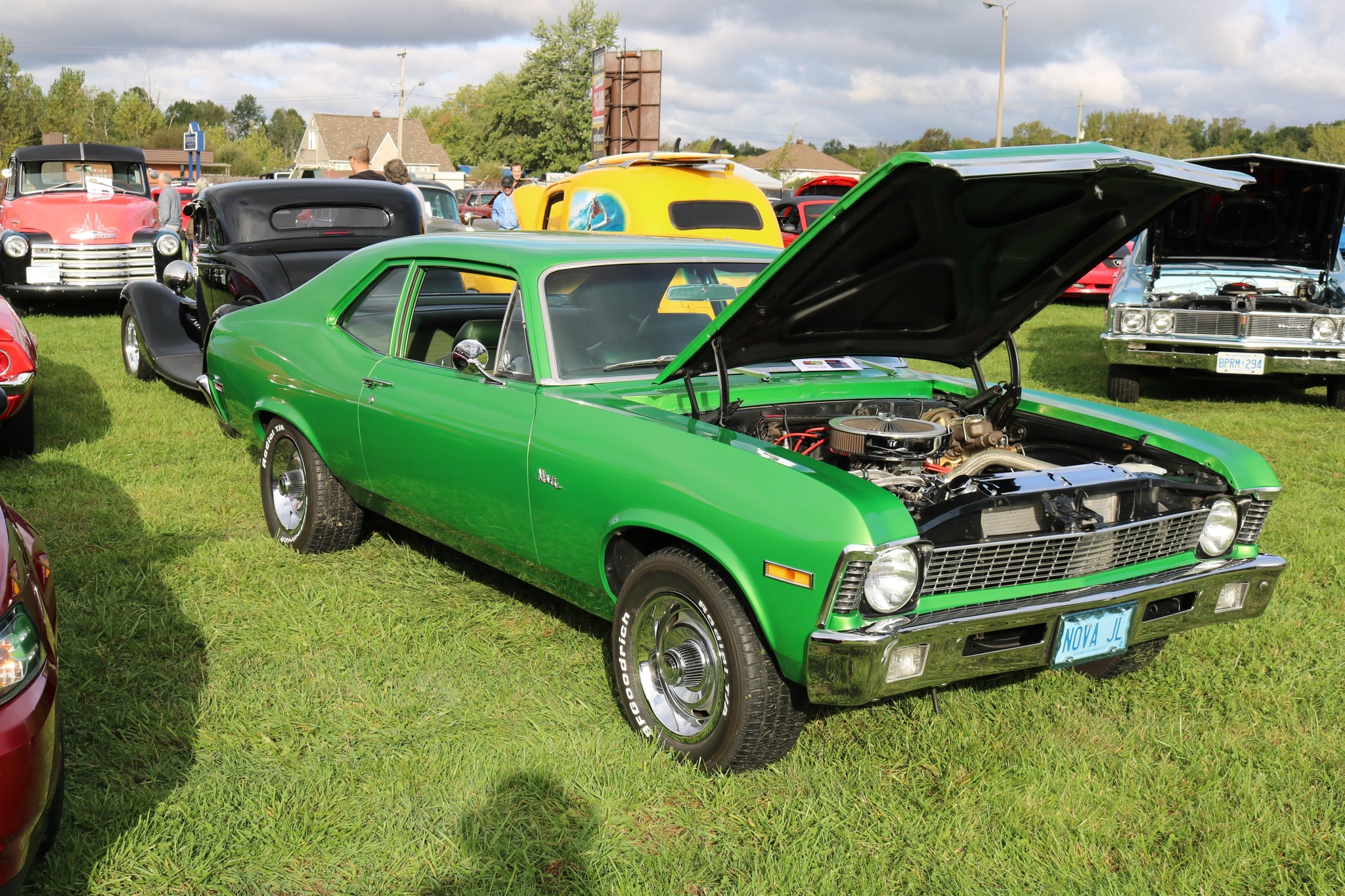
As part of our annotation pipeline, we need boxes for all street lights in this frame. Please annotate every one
[398,82,427,160]
[981,1,1007,149]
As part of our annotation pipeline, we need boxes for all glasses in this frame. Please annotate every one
[349,156,356,160]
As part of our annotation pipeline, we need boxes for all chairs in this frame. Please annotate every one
[595,312,713,362]
[456,319,574,374]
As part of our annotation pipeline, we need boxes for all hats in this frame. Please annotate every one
[501,175,513,187]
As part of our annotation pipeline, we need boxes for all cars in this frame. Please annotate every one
[1058,155,1345,409]
[195,145,1286,777]
[0,494,62,892]
[2,141,183,308]
[1,294,36,461]
[119,152,860,394]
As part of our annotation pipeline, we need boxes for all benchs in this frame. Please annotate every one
[349,306,591,367]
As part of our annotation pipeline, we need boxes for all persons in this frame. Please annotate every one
[510,162,531,190]
[158,172,182,235]
[193,178,209,193]
[491,176,521,231]
[330,145,388,226]
[585,196,610,230]
[384,158,427,232]
